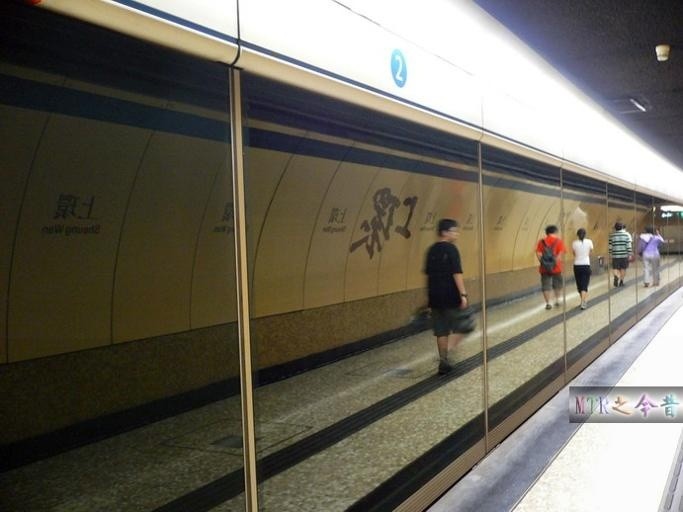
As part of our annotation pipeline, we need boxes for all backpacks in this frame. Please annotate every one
[540,238,559,273]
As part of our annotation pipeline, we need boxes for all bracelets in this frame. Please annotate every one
[460,293,468,297]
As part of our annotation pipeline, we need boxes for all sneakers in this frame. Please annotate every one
[614,276,624,287]
[438,353,457,373]
[546,300,587,310]
[644,282,660,287]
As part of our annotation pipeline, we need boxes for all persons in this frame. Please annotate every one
[572,228,595,310]
[609,222,633,288]
[638,224,665,287]
[535,224,567,310]
[420,218,478,374]
